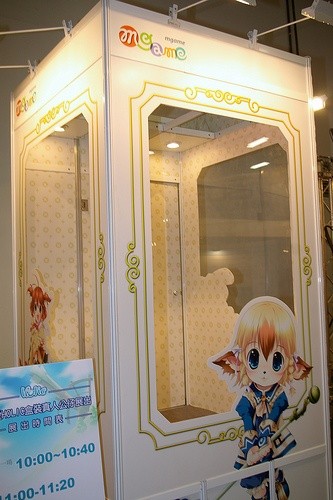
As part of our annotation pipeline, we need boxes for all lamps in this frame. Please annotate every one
[168,0,258,26]
[245,1,333,49]
